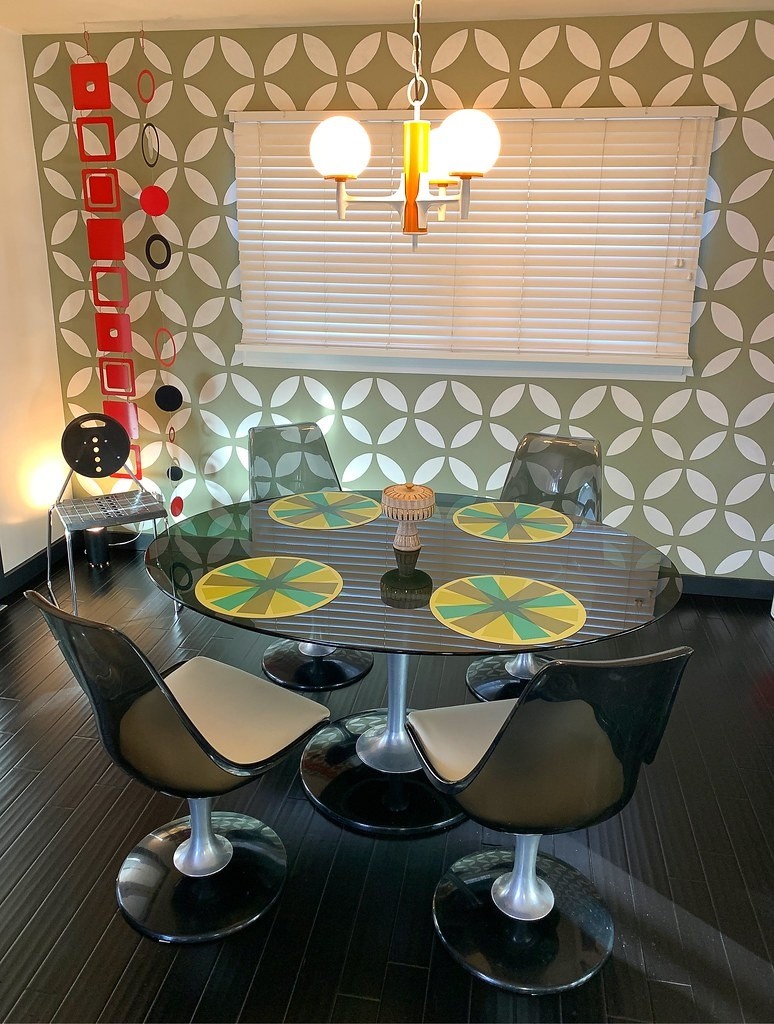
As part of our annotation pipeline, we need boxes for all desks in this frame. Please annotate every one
[144,491,682,839]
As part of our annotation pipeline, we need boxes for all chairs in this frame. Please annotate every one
[24,590,331,945]
[405,646,695,996]
[47,414,184,616]
[466,432,602,702]
[248,423,374,691]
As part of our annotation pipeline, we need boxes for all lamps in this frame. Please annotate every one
[309,1,500,248]
[83,527,110,568]
